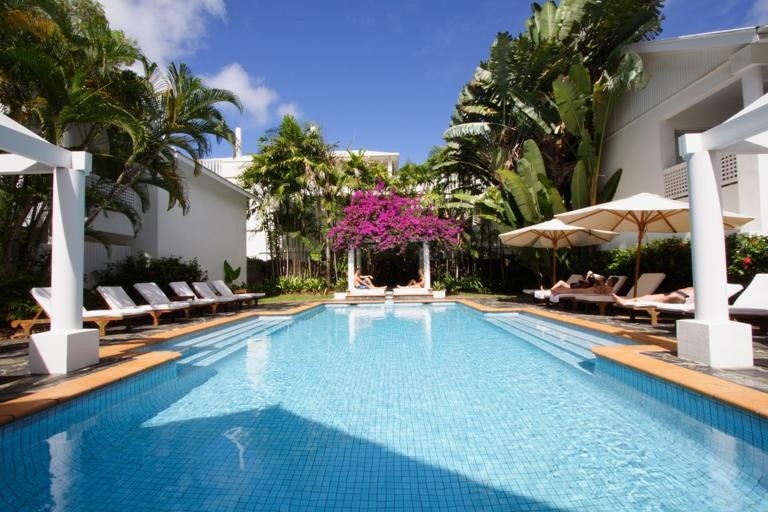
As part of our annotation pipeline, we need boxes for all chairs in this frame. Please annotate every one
[8,276,267,338]
[392,275,426,294]
[518,268,768,345]
[344,273,386,295]
[347,308,386,343]
[394,308,432,345]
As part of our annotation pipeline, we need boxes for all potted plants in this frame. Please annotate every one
[333,285,347,300]
[430,280,446,299]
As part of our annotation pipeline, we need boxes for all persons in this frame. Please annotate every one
[354,268,375,288]
[551,273,619,295]
[610,286,694,306]
[397,268,425,288]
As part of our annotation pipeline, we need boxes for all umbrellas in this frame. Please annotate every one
[554,191,756,301]
[498,218,620,288]
